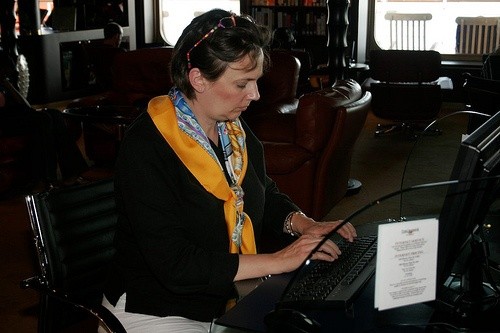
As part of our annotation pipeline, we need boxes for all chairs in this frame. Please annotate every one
[456,17,500,54]
[463,72,500,135]
[385,13,432,51]
[14,177,127,333]
[274,49,323,98]
[364,50,443,144]
[69,45,114,96]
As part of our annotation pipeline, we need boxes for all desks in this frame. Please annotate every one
[63,105,145,180]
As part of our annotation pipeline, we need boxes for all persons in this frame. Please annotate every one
[92,23,127,90]
[0,73,113,184]
[98,9,358,333]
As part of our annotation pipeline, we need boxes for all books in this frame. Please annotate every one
[251,0,327,36]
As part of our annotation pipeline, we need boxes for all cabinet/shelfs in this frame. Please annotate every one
[240,0,358,64]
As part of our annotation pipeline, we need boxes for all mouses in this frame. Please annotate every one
[264,310,319,333]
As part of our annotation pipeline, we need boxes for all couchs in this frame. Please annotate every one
[0,47,373,221]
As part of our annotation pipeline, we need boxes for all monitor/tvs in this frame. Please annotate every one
[423,113,500,327]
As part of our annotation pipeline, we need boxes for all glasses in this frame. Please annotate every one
[185,14,256,72]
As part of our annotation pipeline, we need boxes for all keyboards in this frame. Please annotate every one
[281,236,380,306]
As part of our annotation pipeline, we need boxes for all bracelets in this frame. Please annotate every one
[284,211,306,237]
